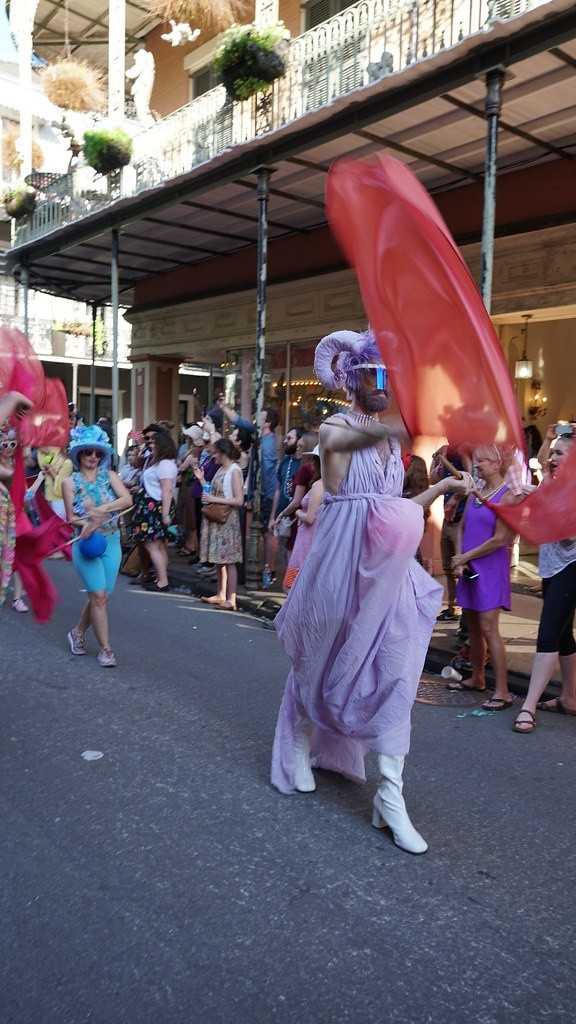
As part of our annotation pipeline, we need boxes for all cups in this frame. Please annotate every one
[441,666,462,682]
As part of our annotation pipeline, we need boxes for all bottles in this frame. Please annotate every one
[262,563,271,590]
[202,481,212,504]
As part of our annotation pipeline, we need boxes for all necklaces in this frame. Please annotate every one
[476,482,506,504]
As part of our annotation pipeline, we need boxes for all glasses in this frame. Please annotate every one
[143,434,157,440]
[79,449,104,459]
[283,435,297,441]
[1,440,17,450]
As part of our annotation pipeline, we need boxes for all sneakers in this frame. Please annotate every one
[68,629,86,654]
[97,649,118,667]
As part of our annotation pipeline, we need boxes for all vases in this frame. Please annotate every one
[221,50,284,101]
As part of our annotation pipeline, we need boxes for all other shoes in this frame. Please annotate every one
[529,585,543,592]
[49,550,66,559]
[128,543,291,630]
[12,598,29,612]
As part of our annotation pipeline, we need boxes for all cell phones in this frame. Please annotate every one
[555,424,573,435]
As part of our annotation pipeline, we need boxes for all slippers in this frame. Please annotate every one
[436,607,576,733]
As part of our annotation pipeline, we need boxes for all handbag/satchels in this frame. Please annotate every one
[276,516,292,537]
[202,466,244,525]
[119,543,143,578]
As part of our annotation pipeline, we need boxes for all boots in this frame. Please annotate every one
[291,713,316,793]
[372,753,429,855]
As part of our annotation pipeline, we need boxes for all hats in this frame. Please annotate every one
[301,442,320,457]
[68,426,113,467]
[181,425,205,446]
[141,419,175,434]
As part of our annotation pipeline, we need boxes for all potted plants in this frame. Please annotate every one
[0,185,36,218]
[82,129,132,177]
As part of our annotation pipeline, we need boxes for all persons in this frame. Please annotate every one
[0,391,113,612]
[61,425,133,666]
[270,330,478,852]
[402,406,576,733]
[120,397,324,630]
[125,49,154,128]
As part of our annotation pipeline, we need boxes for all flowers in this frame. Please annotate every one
[209,20,289,102]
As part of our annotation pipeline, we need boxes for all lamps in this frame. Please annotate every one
[515,313,534,380]
[527,377,547,421]
